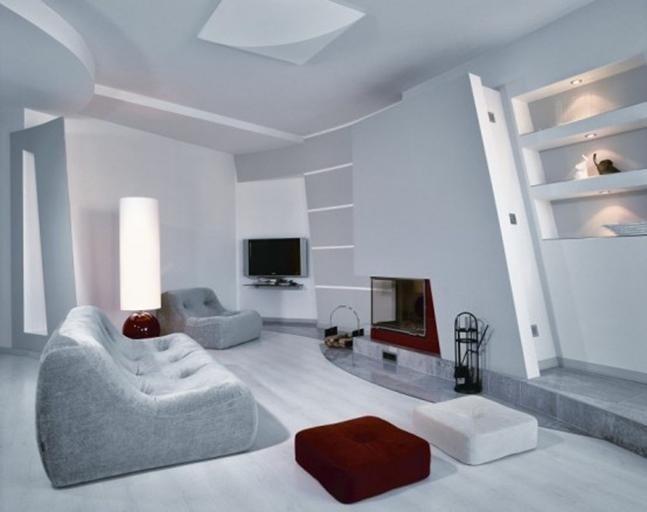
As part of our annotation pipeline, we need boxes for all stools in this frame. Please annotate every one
[295,416,430,504]
[413,395,538,466]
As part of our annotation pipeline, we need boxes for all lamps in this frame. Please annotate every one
[118,195,162,339]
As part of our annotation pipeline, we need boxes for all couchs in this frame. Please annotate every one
[156,288,262,350]
[34,305,257,489]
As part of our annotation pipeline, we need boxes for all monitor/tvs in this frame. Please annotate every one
[372,276,426,337]
[243,238,307,290]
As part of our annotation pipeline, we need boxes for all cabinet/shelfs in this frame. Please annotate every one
[504,31,647,241]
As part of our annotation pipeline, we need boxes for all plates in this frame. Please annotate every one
[602,222,647,237]
